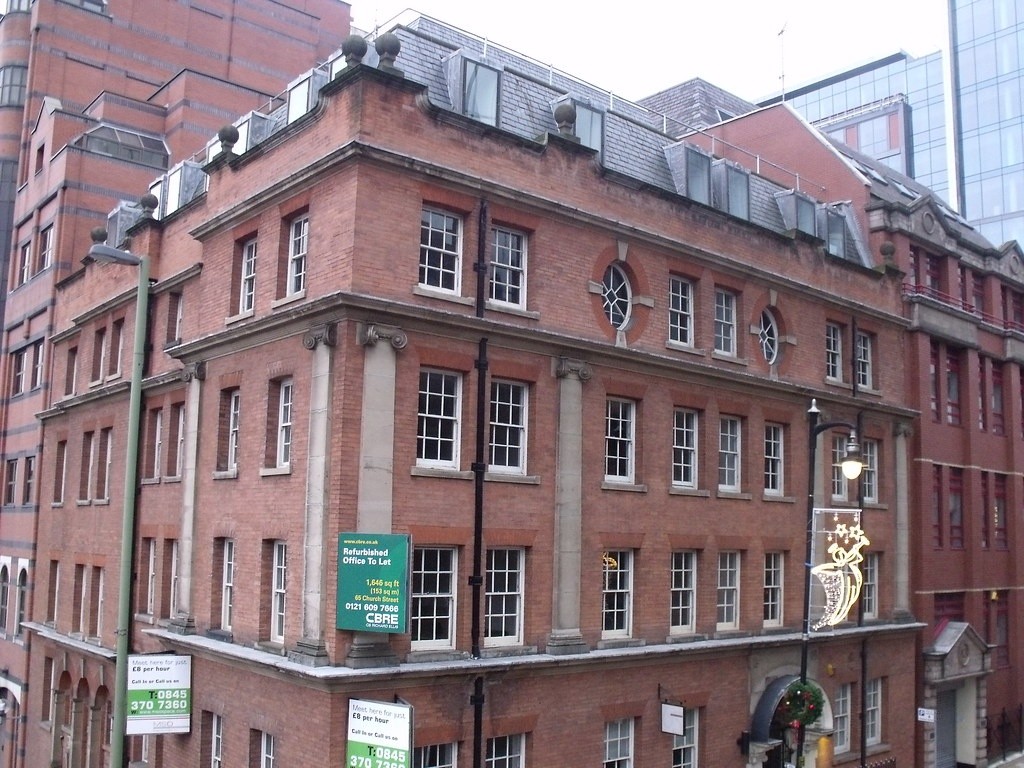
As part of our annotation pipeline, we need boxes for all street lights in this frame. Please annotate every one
[796,399,863,768]
[88,244,151,768]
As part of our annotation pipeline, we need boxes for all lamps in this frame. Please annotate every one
[810,417,861,481]
